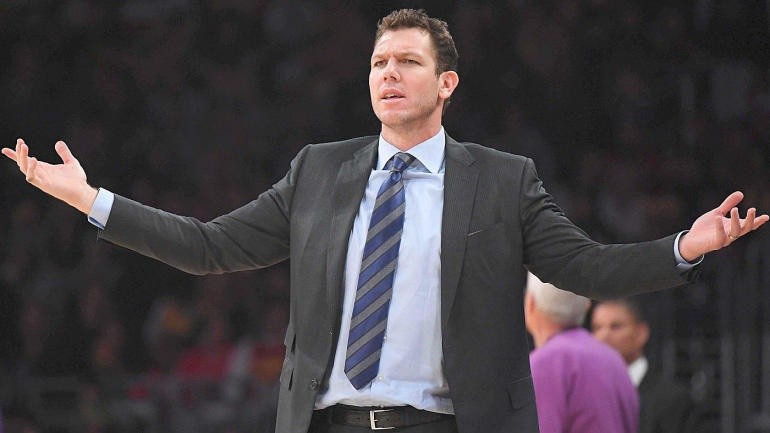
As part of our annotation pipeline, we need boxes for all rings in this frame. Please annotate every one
[727,232,738,241]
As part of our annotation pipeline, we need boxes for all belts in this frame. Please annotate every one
[313,406,455,429]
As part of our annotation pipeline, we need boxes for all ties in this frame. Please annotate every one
[344,153,416,390]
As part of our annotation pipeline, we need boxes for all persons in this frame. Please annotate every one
[525,271,642,433]
[2,9,770,433]
[591,298,685,433]
